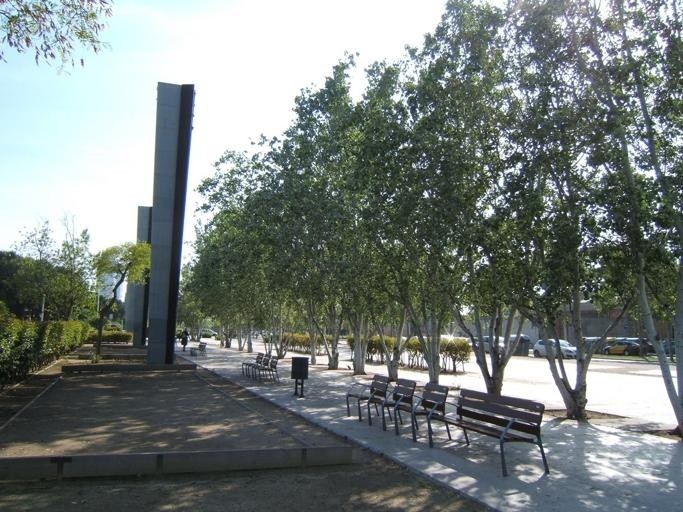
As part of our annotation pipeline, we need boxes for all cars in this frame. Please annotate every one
[534,339,578,358]
[605,340,640,355]
[195,329,218,336]
[467,337,478,349]
[483,336,505,353]
[509,334,533,349]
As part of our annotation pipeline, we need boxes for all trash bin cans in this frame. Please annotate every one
[292,357,309,398]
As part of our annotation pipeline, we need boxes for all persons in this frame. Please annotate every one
[178,327,191,352]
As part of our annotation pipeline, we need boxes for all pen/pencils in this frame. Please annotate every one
[504,334,530,356]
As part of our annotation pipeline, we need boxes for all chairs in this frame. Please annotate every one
[190,341,207,356]
[242,353,280,382]
[346,375,549,476]
